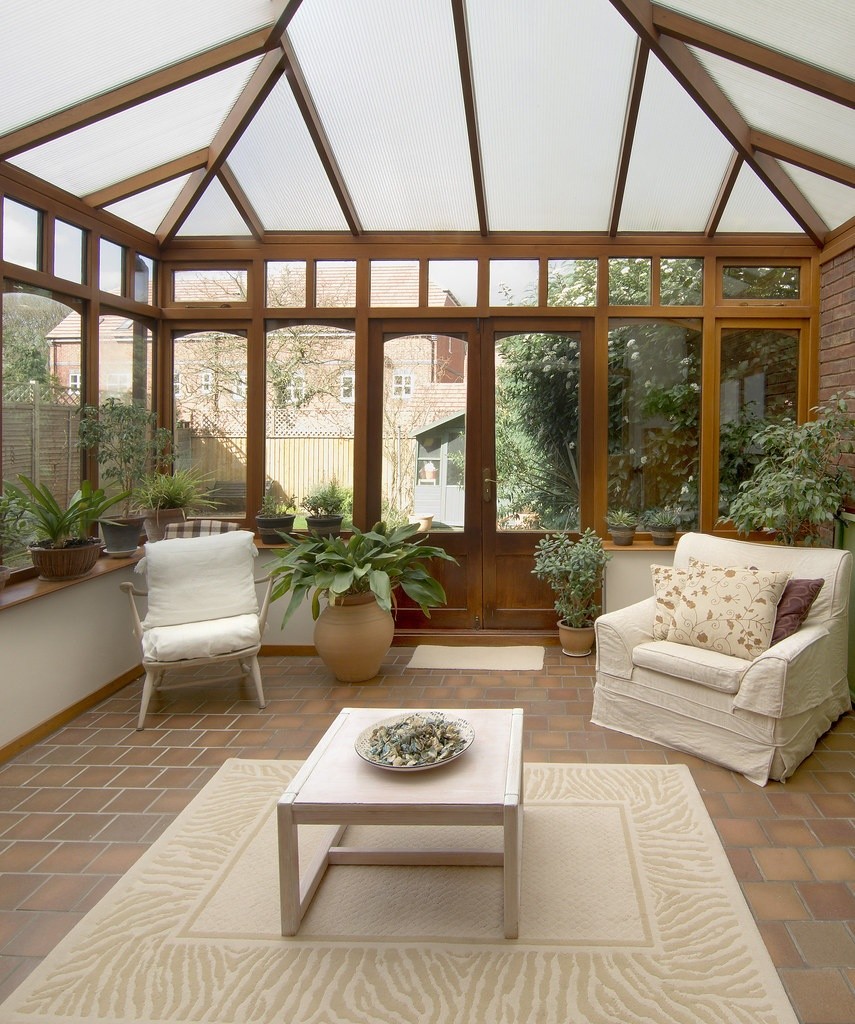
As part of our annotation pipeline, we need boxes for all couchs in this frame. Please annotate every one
[590,533,852,787]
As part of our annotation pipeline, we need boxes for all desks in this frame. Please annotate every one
[418,479,436,485]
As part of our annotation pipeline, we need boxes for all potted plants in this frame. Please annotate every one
[255,493,298,544]
[130,462,226,543]
[602,508,639,546]
[420,462,437,479]
[260,517,460,682]
[639,506,684,546]
[299,482,352,541]
[1,473,131,581]
[0,491,32,592]
[530,527,614,656]
[70,397,184,559]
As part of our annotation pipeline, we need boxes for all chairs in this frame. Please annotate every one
[119,520,275,731]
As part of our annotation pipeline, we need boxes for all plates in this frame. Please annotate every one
[355,711,474,772]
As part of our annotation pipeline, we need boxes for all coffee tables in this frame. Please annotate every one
[277,708,524,939]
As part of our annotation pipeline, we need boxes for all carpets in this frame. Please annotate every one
[0,757,800,1024]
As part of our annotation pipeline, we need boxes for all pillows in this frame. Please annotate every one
[134,531,259,630]
[770,578,825,648]
[651,563,758,641]
[667,556,793,662]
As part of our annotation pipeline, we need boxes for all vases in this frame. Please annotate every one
[409,513,434,531]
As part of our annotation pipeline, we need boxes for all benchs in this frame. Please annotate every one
[205,481,275,510]
